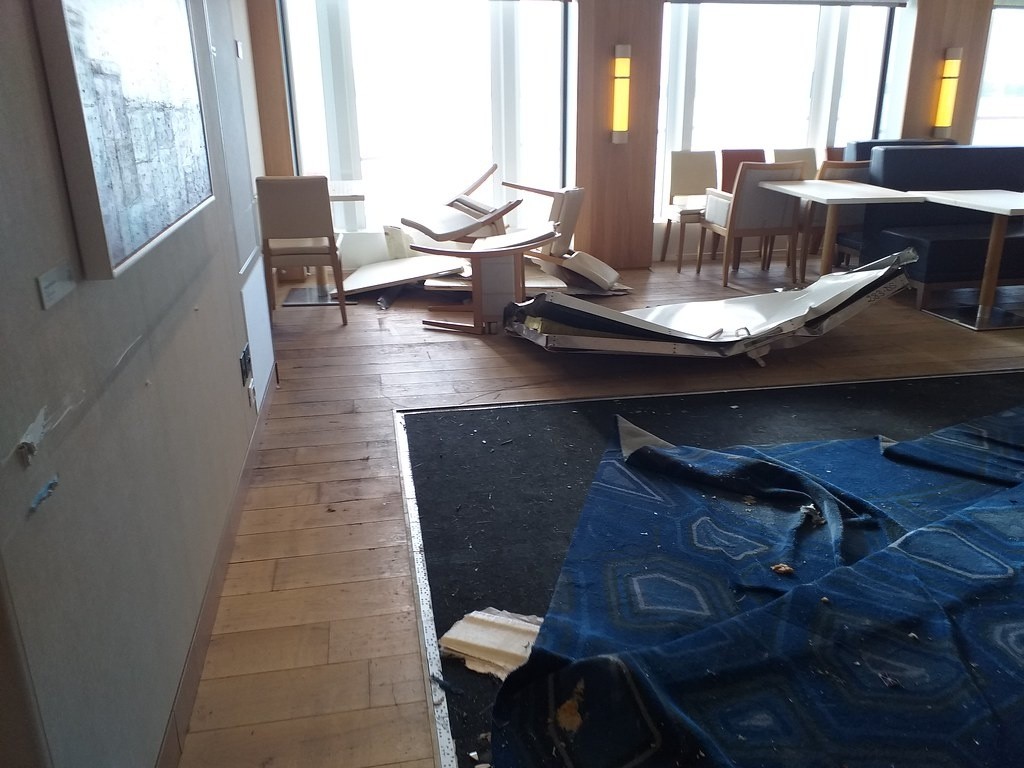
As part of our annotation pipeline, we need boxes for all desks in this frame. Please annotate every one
[910,186,1024,334]
[759,178,922,289]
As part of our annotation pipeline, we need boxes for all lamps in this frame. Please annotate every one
[608,39,632,147]
[934,43,965,138]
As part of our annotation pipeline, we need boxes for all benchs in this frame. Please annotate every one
[840,140,1024,313]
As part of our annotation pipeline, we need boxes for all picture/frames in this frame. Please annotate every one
[35,0,220,289]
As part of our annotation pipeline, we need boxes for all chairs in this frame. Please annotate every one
[657,149,738,274]
[825,146,843,161]
[251,158,624,338]
[720,146,775,274]
[695,158,808,288]
[771,145,818,263]
[798,158,872,278]
